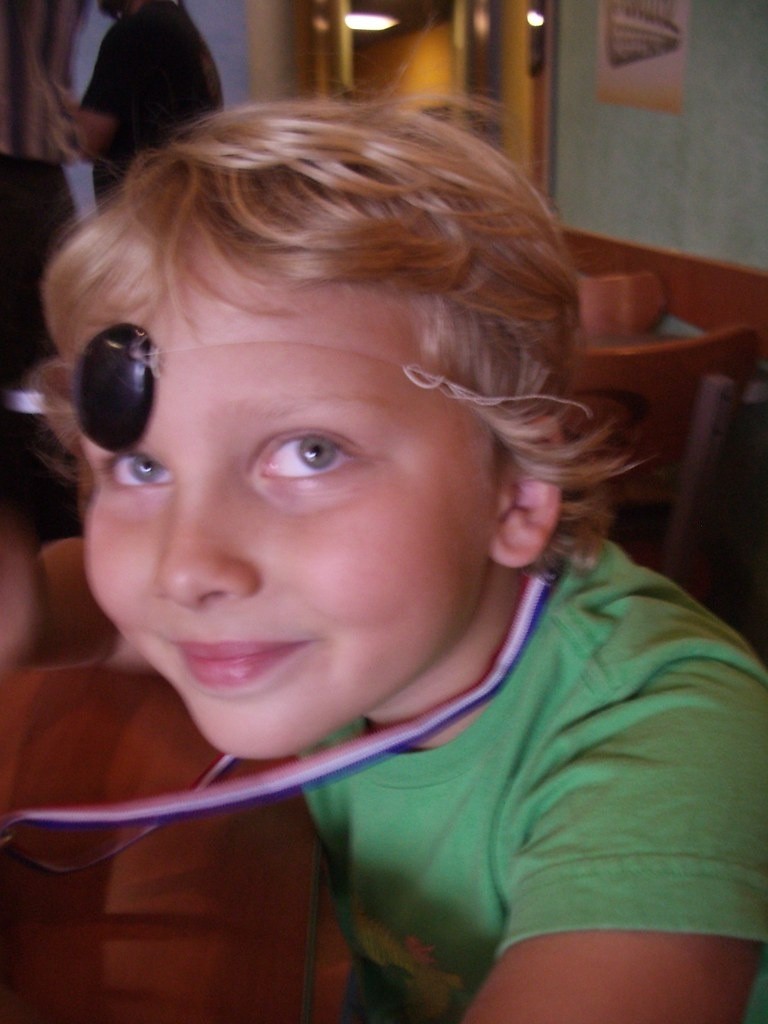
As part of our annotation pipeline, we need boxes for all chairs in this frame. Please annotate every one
[568,324,761,602]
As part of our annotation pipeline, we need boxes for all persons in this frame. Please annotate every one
[0,101,768,1024]
[0,0,222,542]
